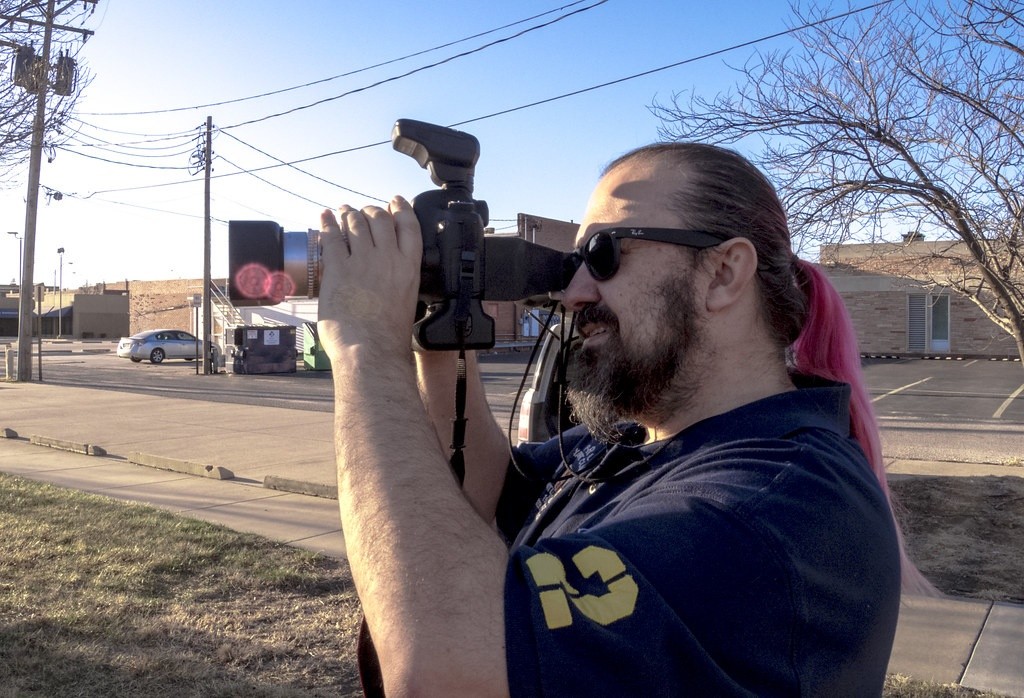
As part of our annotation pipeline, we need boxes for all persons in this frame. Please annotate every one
[317,141,901,698]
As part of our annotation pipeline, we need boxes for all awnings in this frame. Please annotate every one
[32,305,54,317]
[0,309,19,318]
[46,306,73,318]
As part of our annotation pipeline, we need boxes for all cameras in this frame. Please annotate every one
[228,117,578,353]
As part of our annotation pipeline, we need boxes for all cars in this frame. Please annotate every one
[116,327,221,364]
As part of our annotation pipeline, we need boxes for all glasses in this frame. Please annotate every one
[570,228,770,282]
